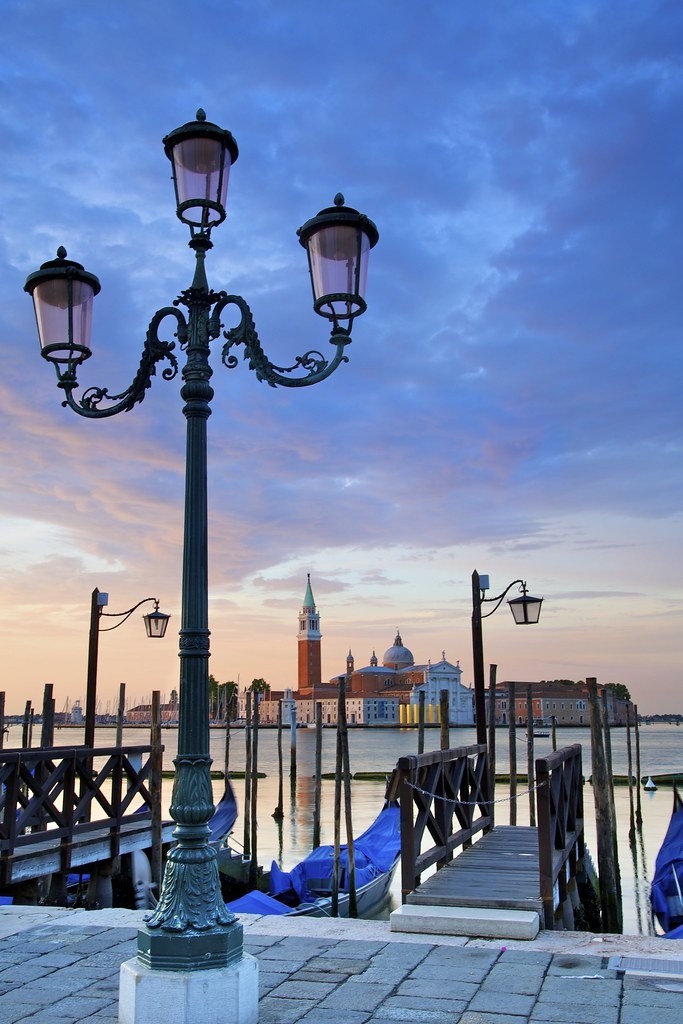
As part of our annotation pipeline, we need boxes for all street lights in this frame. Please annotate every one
[471,569,539,808]
[23,101,382,972]
[83,586,171,792]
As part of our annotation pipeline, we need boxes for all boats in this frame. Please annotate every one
[525,731,550,737]
[643,776,658,791]
